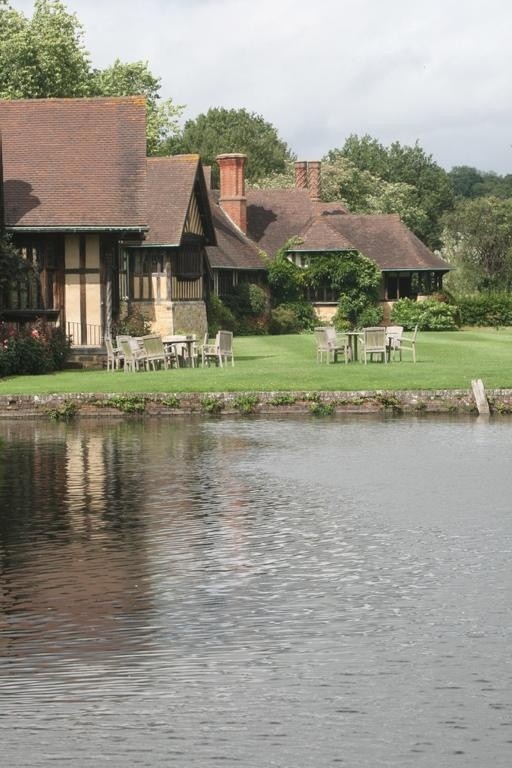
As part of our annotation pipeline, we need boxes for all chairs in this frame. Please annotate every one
[314,323,419,365]
[104,330,234,371]
